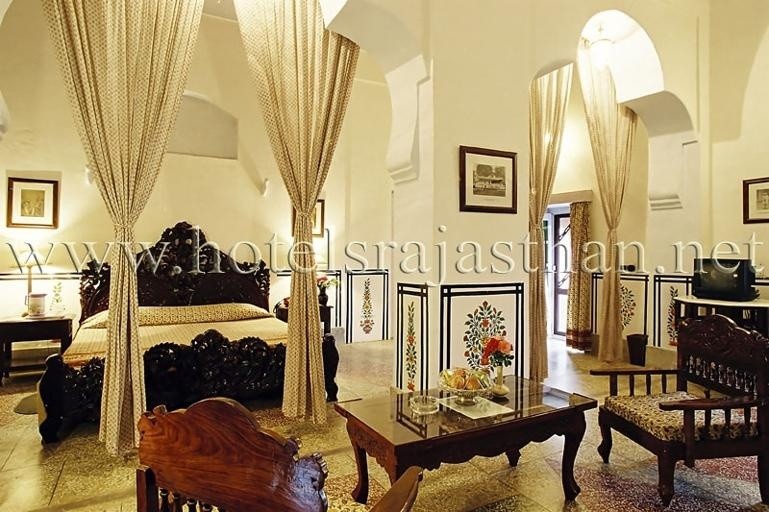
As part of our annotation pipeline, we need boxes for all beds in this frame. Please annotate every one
[35,222,340,448]
[334,374,597,504]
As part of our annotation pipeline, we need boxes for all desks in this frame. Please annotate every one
[670,295,769,339]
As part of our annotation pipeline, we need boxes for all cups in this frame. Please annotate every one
[30,298,42,315]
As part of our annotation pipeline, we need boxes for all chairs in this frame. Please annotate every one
[590,314,769,506]
[136,396,423,512]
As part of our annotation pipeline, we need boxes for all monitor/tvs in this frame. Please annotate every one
[692,259,760,302]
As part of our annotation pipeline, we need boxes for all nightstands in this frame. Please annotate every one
[276,297,333,339]
[1,317,72,389]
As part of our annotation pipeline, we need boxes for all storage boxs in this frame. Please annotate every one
[11,339,62,360]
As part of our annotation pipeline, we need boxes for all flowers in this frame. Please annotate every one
[316,274,338,289]
[481,334,514,367]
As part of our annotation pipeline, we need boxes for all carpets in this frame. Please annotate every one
[336,382,362,402]
[14,393,39,414]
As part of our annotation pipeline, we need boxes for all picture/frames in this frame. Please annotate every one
[7,177,58,228]
[292,199,323,236]
[458,146,516,214]
[743,177,769,224]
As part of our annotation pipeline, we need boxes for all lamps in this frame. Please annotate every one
[582,25,612,56]
[8,251,53,317]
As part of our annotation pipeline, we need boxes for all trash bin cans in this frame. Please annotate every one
[627,334,649,367]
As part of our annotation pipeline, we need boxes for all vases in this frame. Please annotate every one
[318,288,330,304]
[491,365,510,396]
